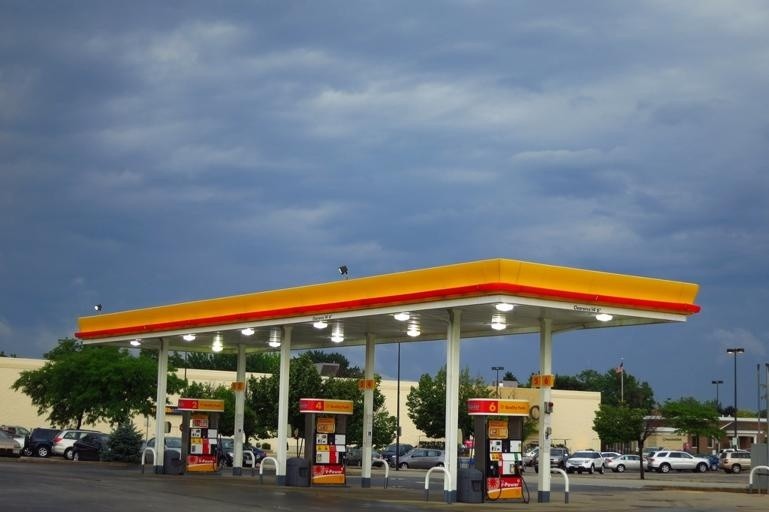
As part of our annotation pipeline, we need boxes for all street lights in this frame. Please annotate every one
[726,347,743,447]
[711,380,724,401]
[491,366,504,398]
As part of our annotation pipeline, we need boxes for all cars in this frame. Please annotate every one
[346,446,382,467]
[218,437,254,468]
[146,434,181,464]
[0,430,21,457]
[243,444,265,463]
[72,433,111,460]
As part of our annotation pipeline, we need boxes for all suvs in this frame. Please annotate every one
[392,447,444,470]
[521,446,753,476]
[0,425,32,456]
[52,428,103,460]
[30,427,61,458]
[381,443,413,463]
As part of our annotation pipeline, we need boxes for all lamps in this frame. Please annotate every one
[339,266,349,280]
[94,304,102,315]
